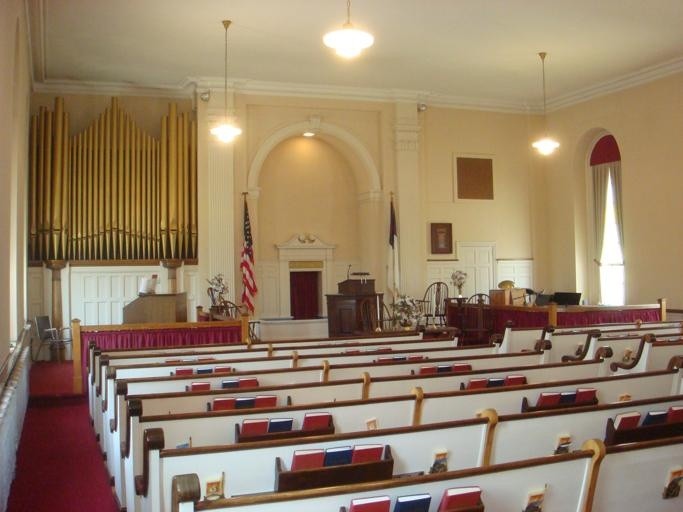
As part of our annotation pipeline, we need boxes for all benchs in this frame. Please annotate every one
[85,320,683,512]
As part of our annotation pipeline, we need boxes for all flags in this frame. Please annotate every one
[387,198,402,298]
[240,195,259,317]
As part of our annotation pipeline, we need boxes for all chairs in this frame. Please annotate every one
[362,297,394,334]
[218,301,260,344]
[33,315,72,366]
[422,281,449,327]
[460,294,494,347]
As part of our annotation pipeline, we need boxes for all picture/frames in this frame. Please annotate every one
[452,153,497,205]
[426,218,458,261]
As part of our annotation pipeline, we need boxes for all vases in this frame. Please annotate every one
[458,285,462,298]
[213,291,221,307]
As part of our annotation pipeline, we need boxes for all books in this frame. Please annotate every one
[439,485,482,512]
[324,445,352,468]
[352,444,384,463]
[290,448,326,470]
[612,409,642,431]
[665,405,683,423]
[213,394,277,411]
[377,355,417,362]
[175,366,234,376]
[378,347,392,351]
[347,494,391,512]
[419,363,472,374]
[467,374,525,389]
[165,356,216,363]
[191,378,257,391]
[241,412,330,436]
[393,492,433,512]
[534,387,597,407]
[346,349,360,353]
[639,410,670,427]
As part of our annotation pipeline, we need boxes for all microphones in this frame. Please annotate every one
[347,264,352,279]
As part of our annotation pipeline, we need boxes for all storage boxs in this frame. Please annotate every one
[489,287,526,305]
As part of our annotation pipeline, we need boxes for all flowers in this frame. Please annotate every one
[391,293,421,321]
[205,272,228,295]
[451,270,467,287]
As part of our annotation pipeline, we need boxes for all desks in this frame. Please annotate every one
[444,297,468,327]
[209,306,231,322]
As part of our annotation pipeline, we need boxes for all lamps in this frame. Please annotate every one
[532,53,561,156]
[209,21,242,145]
[321,0,376,62]
